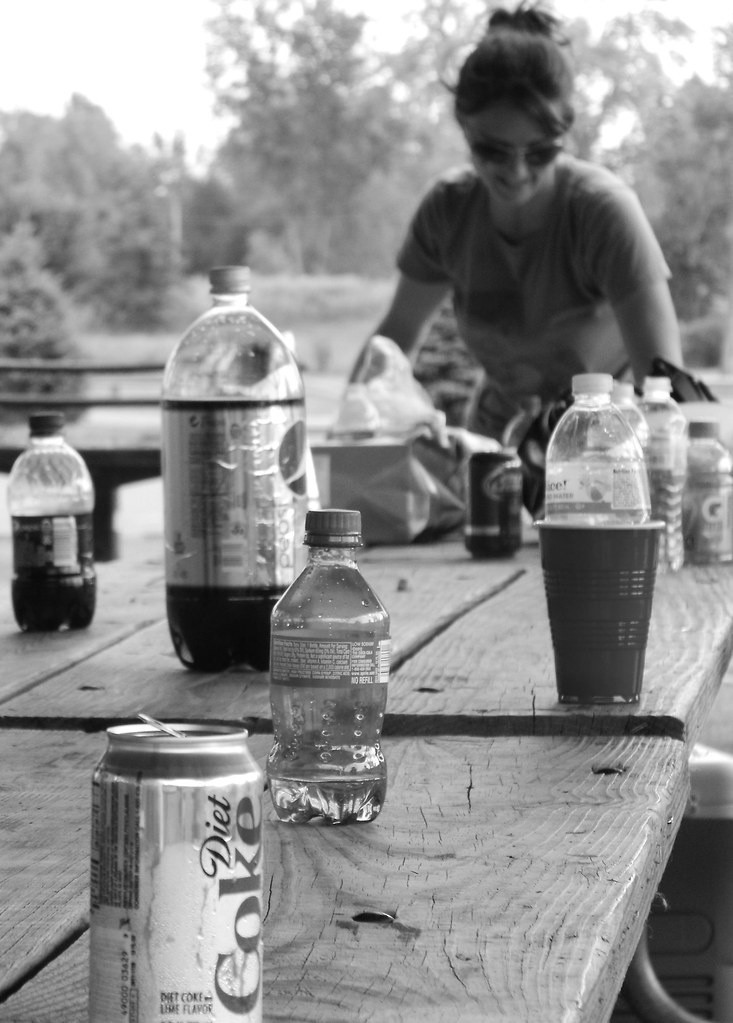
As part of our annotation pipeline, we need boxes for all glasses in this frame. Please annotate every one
[466,140,563,167]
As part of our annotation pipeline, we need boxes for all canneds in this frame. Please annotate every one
[463,448,525,556]
[90,712,266,1023]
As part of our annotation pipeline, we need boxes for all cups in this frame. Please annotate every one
[537,520,665,703]
[310,442,434,544]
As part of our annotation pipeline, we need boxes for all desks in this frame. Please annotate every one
[0,526,732,1023]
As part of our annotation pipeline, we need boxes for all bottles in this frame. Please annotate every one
[268,509,389,825]
[638,375,685,573]
[7,411,97,630]
[683,422,733,564]
[163,266,306,670]
[546,373,653,523]
[502,396,541,457]
[610,380,649,465]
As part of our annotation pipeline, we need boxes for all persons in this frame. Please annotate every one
[350,0,682,444]
